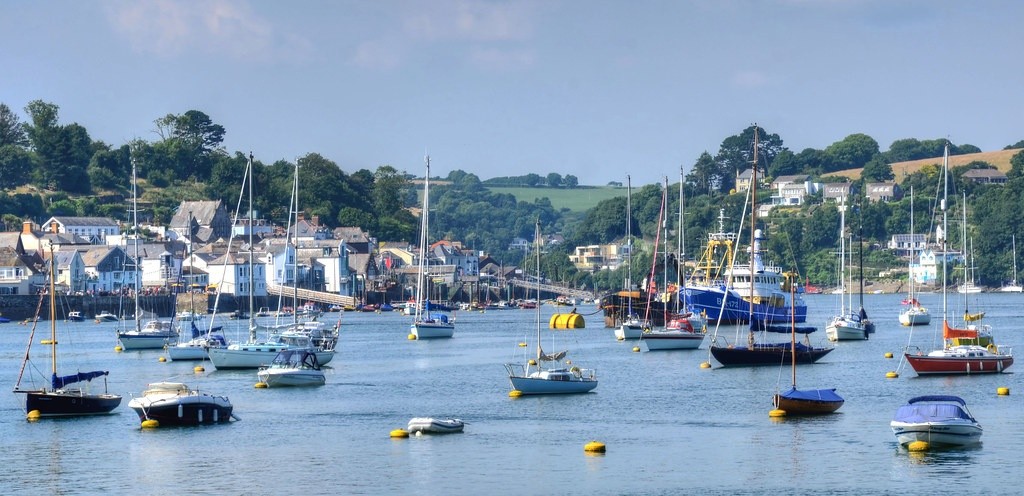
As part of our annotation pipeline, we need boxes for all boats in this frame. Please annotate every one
[0,315,13,324]
[890,394,984,450]
[256,346,328,389]
[66,311,85,323]
[24,315,43,323]
[407,415,464,434]
[175,310,205,322]
[126,380,234,429]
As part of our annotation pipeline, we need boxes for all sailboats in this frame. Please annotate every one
[904,136,1014,378]
[11,238,124,419]
[164,210,231,363]
[406,155,457,340]
[853,226,878,334]
[93,266,118,322]
[501,215,600,395]
[597,162,808,351]
[263,158,341,350]
[955,236,989,295]
[113,157,182,351]
[824,187,868,343]
[201,150,335,371]
[999,235,1024,293]
[896,184,933,328]
[706,120,836,366]
[948,189,994,348]
[770,268,846,415]
[228,233,593,320]
